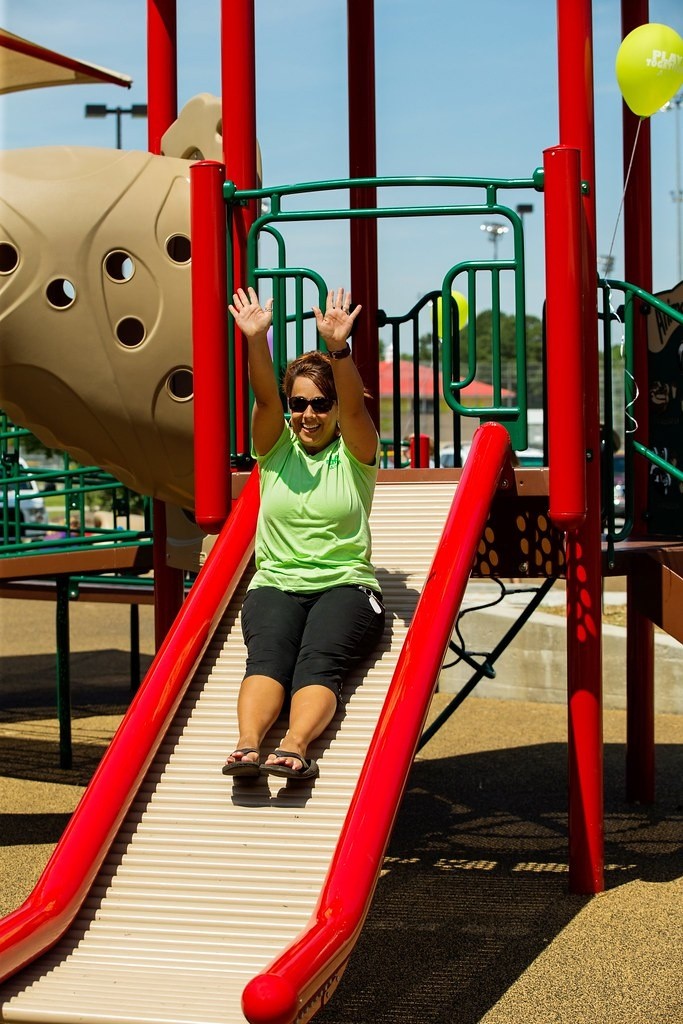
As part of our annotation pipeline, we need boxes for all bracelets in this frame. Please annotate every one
[327,342,351,360]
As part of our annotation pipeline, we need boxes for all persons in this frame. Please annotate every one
[27,512,101,542]
[220,285,386,781]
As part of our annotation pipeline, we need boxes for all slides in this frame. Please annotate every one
[0,414,514,1024]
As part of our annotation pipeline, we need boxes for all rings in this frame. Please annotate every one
[343,308,349,312]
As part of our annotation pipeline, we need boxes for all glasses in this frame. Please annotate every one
[288,396,335,413]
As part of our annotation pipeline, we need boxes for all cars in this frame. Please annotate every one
[0,456,48,538]
[437,442,544,466]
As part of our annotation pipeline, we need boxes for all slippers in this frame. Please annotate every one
[259,750,319,779]
[222,748,262,776]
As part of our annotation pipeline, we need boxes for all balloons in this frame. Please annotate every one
[614,23,683,116]
[431,291,468,337]
